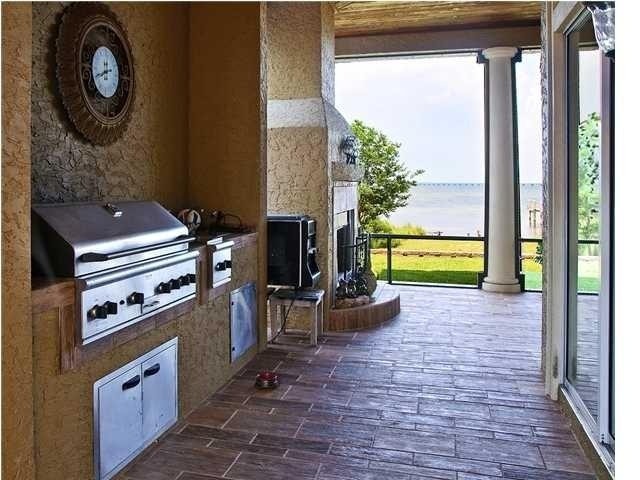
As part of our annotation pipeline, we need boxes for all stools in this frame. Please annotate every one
[269,288,326,353]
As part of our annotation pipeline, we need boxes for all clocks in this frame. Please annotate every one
[50,2,136,148]
